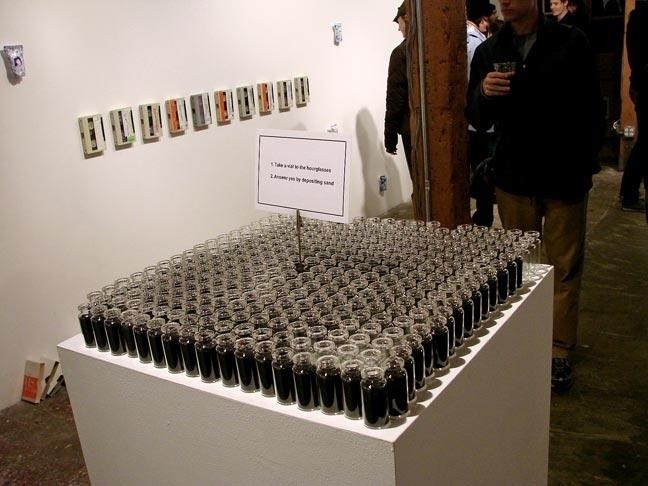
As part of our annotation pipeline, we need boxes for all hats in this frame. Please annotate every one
[393,2,406,24]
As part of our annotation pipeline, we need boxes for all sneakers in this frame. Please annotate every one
[551,349,573,392]
[622,198,647,213]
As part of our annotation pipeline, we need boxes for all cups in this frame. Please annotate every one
[495,61,515,81]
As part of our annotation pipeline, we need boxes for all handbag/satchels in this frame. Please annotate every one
[472,157,495,199]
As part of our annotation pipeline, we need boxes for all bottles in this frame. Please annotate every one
[77,214,541,429]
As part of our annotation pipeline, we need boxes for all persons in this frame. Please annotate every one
[466,0,577,228]
[385,0,411,179]
[466,1,603,394]
[619,0,648,212]
